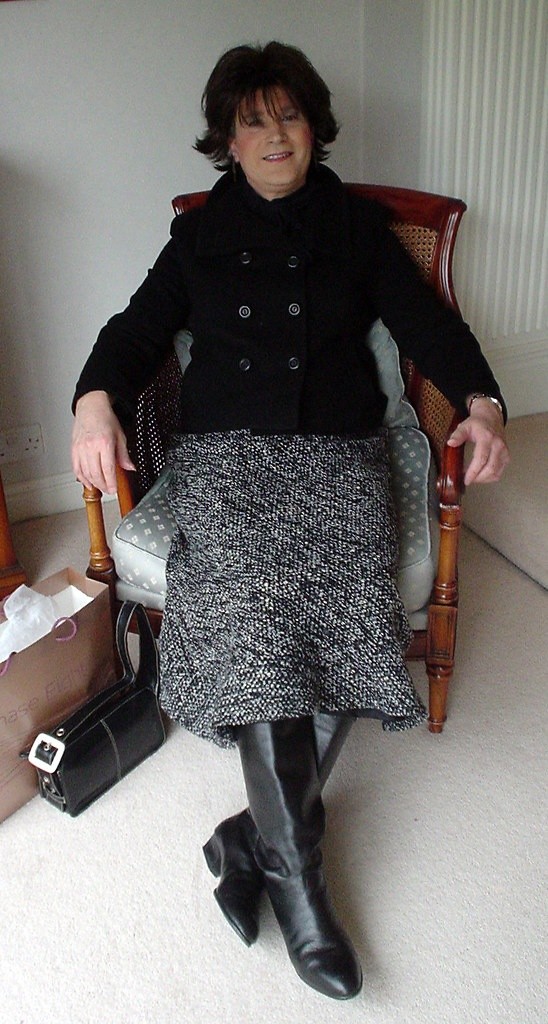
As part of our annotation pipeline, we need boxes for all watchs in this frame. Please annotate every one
[467,394,502,418]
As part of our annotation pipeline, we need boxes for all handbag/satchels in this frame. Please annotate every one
[0,567,118,824]
[20,599,166,818]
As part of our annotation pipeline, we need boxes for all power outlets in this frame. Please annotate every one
[0,423,43,466]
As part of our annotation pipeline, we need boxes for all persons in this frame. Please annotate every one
[71,42,510,1001]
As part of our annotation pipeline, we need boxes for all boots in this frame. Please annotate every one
[202,711,356,947]
[235,715,363,1000]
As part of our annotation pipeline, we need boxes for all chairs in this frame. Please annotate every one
[80,181,472,733]
